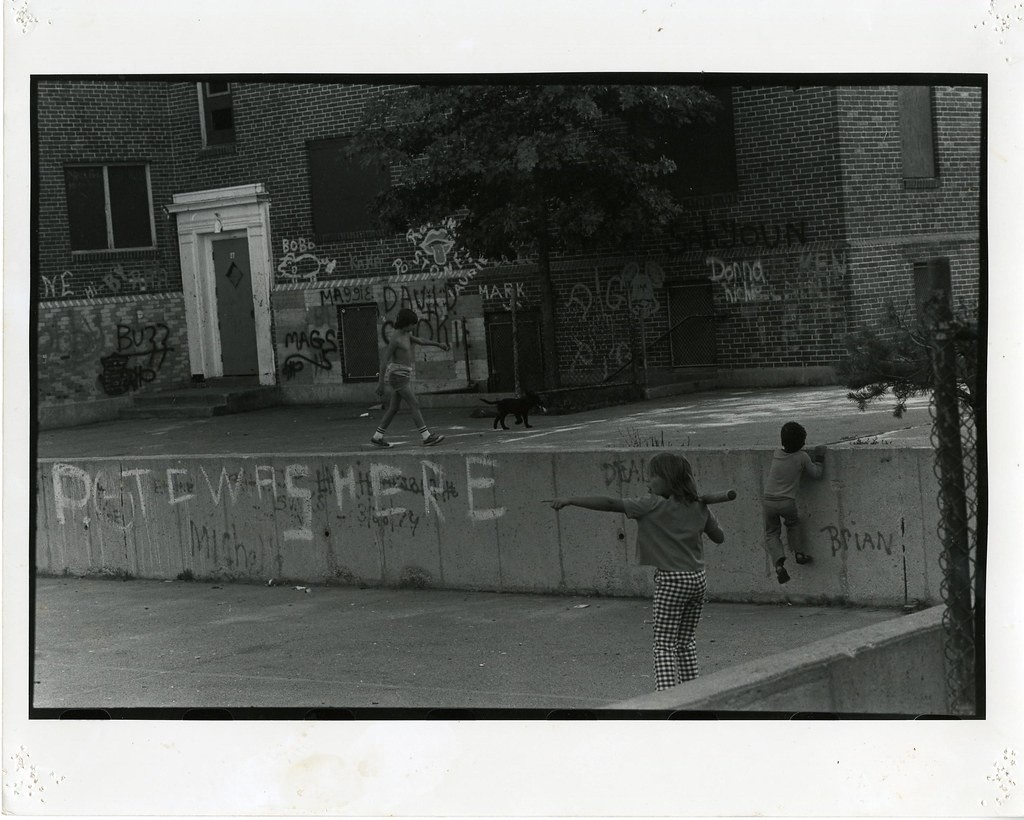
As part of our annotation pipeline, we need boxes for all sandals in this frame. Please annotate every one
[795,553,812,564]
[775,564,790,584]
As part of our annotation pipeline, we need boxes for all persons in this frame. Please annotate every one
[370,308,449,448]
[541,453,725,693]
[763,421,827,585]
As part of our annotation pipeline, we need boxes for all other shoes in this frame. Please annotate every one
[423,433,445,446]
[371,436,393,448]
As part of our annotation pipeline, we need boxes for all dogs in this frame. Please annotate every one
[480,396,540,430]
